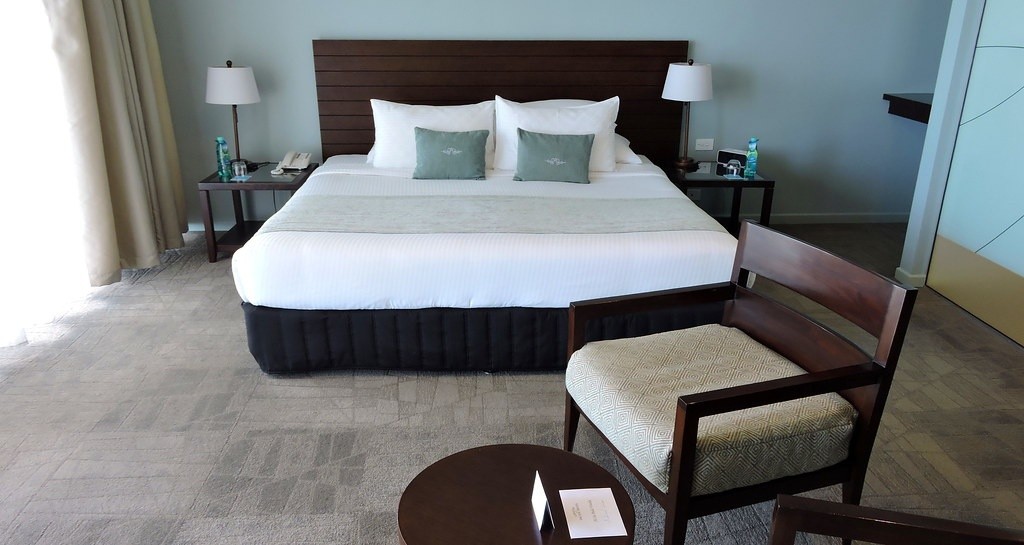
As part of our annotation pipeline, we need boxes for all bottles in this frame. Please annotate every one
[215,137,231,176]
[744,136,759,177]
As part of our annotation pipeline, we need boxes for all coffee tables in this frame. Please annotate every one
[198,161,320,263]
[675,165,775,233]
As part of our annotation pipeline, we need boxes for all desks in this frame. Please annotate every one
[883,92,935,124]
[398,444,635,545]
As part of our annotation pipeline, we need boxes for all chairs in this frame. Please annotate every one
[564,219,920,545]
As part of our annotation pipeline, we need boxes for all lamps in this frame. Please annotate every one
[206,60,261,169]
[661,58,714,173]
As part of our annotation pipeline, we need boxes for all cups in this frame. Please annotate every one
[726,160,741,177]
[232,162,247,180]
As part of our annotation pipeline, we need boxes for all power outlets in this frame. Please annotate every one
[687,189,701,201]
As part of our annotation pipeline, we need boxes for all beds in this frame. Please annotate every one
[231,40,756,374]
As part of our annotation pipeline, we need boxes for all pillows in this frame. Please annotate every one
[492,95,620,174]
[513,127,595,185]
[367,97,494,170]
[520,99,642,165]
[412,126,489,180]
